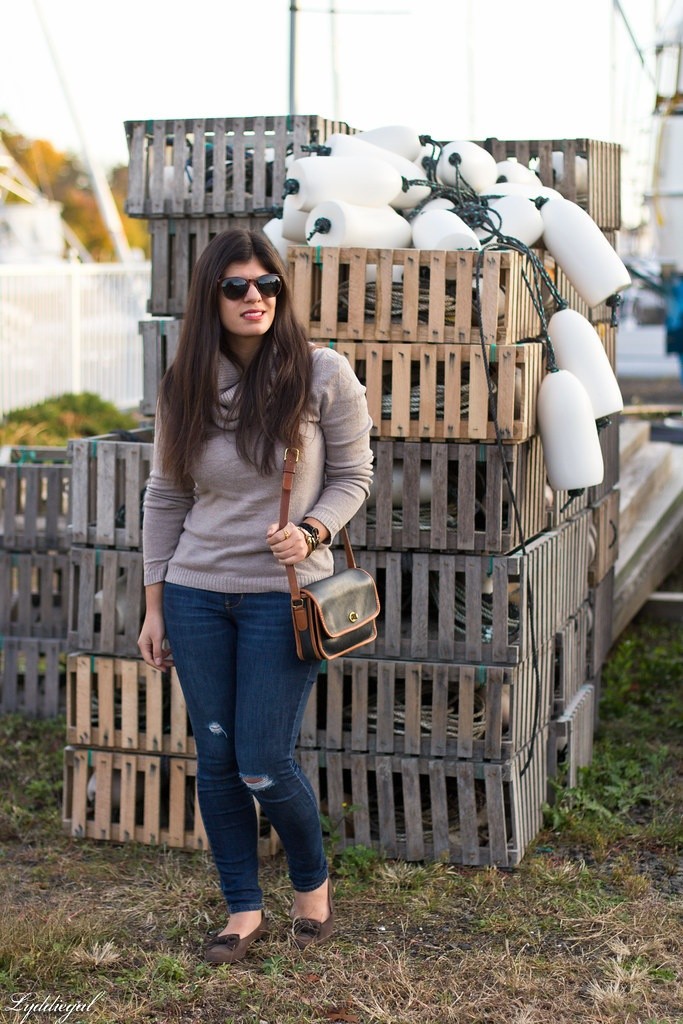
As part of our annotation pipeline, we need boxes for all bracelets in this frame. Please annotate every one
[297,526,316,558]
[298,523,319,551]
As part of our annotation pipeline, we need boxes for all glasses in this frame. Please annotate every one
[214,273,282,301]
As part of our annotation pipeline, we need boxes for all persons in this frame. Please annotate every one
[133,227,383,966]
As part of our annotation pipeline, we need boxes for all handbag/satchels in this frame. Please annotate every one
[291,565,382,662]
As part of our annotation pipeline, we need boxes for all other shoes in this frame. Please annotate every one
[290,877,335,948]
[205,909,268,964]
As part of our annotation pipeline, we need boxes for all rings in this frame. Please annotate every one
[282,528,289,539]
